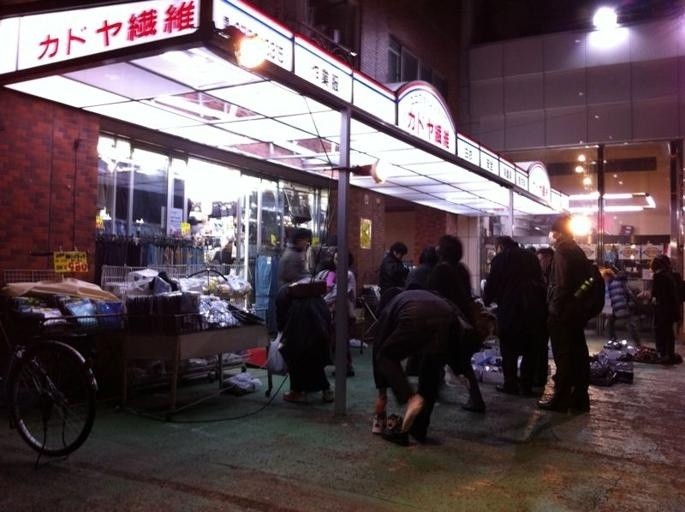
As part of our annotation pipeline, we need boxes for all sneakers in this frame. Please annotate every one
[319,390,333,402]
[372,413,427,444]
[461,401,486,411]
[283,391,306,402]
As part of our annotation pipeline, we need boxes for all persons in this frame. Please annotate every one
[537,218,590,414]
[372,286,470,443]
[536,247,558,388]
[274,227,311,288]
[483,236,547,398]
[642,255,684,364]
[415,246,439,290]
[379,241,410,297]
[604,245,619,264]
[323,249,357,376]
[274,283,334,402]
[525,246,537,254]
[312,256,337,324]
[598,261,641,346]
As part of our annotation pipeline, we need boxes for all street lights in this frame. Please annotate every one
[330,160,386,419]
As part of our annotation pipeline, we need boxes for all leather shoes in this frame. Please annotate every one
[496,385,504,391]
[536,401,551,409]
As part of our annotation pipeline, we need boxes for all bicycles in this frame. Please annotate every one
[0,311,103,472]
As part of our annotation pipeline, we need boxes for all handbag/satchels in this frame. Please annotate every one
[564,246,605,318]
[472,296,496,342]
[289,277,327,295]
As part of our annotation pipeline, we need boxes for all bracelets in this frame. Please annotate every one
[379,395,387,399]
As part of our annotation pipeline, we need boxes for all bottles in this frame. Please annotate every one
[573,276,594,298]
